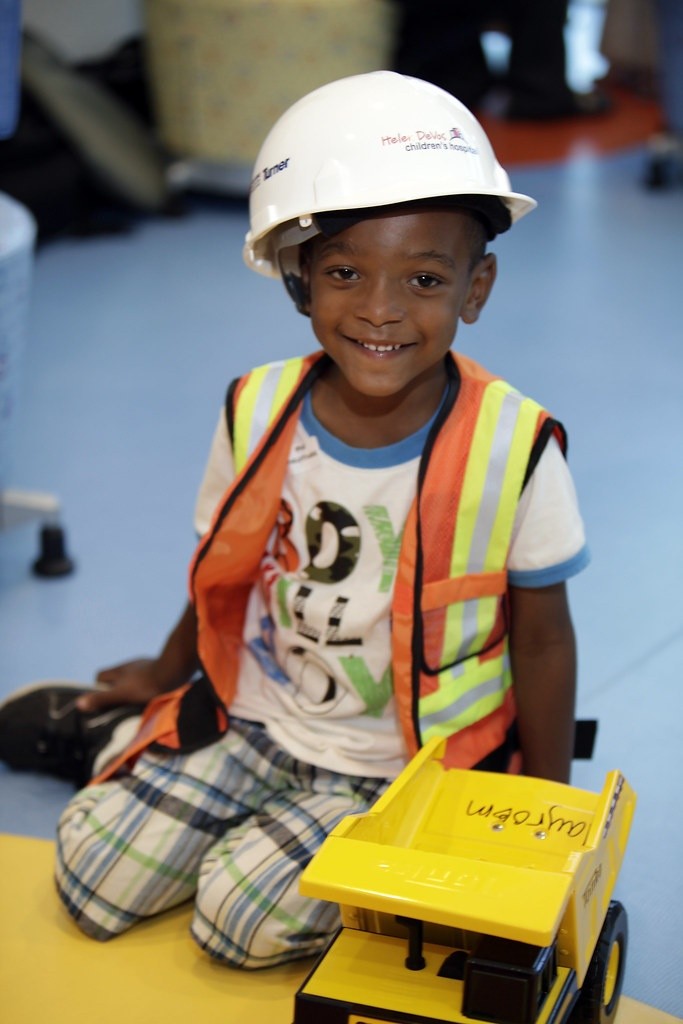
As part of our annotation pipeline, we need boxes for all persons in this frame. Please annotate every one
[0,70,590,969]
[396,0,608,128]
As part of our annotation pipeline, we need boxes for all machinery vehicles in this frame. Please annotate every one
[293,738,637,1024]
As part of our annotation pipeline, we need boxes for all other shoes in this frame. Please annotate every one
[639,145,683,190]
[505,87,611,123]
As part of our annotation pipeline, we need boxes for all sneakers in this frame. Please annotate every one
[0,683,149,793]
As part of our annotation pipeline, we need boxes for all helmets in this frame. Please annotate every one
[243,72,538,317]
